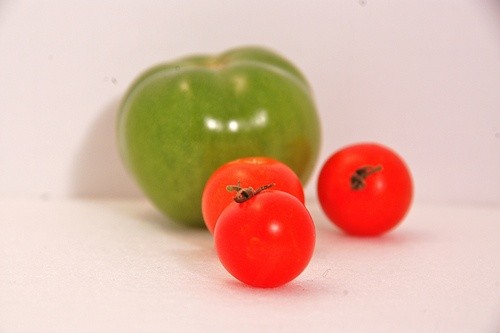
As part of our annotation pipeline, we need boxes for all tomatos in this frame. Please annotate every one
[213,180,318,289]
[201,155,304,233]
[317,143,414,237]
